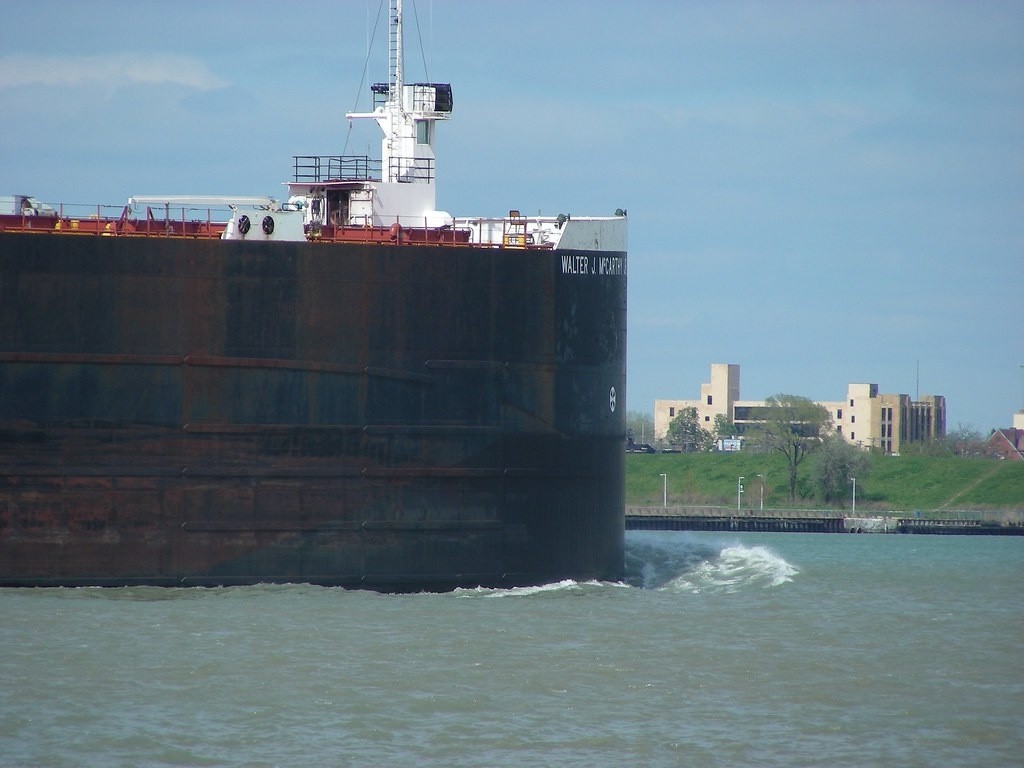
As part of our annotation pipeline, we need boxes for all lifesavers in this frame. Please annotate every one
[237,214,251,235]
[262,215,274,233]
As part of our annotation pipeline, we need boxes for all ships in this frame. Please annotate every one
[1,1,630,596]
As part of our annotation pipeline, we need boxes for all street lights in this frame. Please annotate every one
[757,474,764,509]
[851,478,856,512]
[739,477,744,509]
[660,474,667,506]
